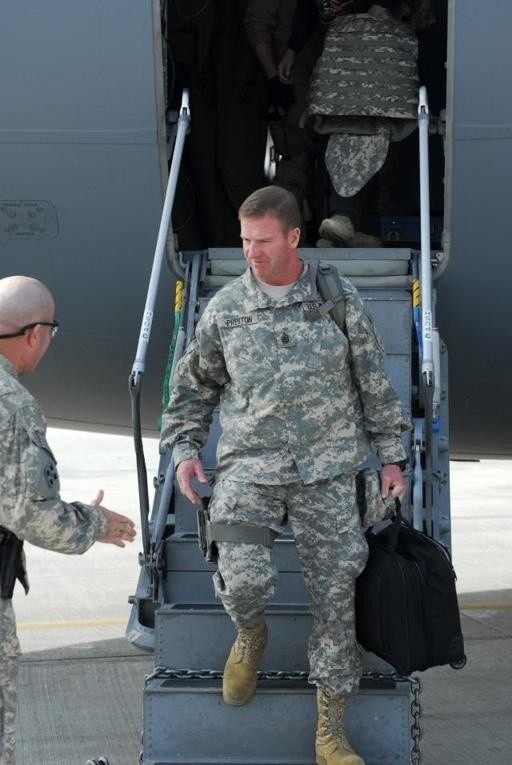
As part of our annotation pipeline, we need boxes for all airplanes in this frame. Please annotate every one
[0,0,511,765]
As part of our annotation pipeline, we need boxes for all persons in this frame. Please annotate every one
[160,185,413,765]
[241,0,383,248]
[0,275,136,765]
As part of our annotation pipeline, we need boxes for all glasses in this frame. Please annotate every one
[18,320,59,337]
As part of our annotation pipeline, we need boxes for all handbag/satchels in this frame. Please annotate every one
[353,488,474,680]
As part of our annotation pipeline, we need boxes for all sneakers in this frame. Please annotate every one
[319,213,355,249]
[314,230,384,248]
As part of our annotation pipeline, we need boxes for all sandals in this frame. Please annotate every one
[220,614,268,708]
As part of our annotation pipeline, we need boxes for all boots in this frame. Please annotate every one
[312,688,366,765]
